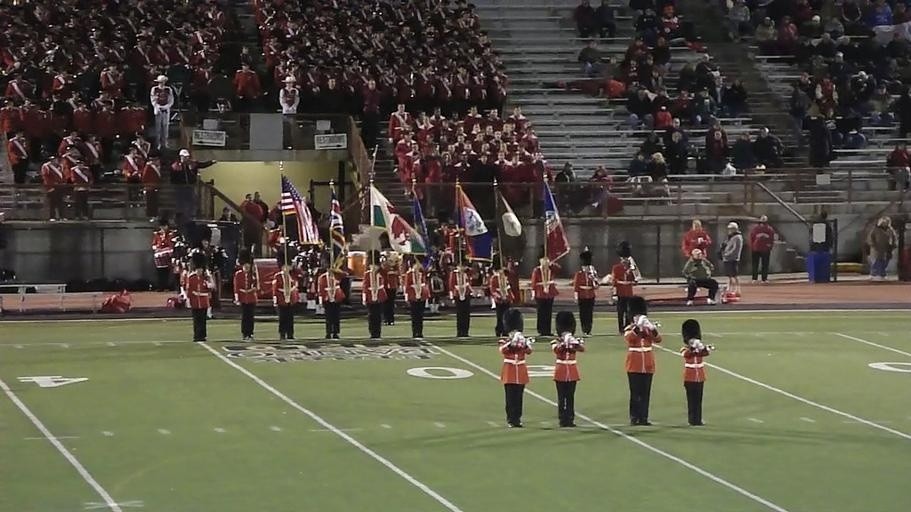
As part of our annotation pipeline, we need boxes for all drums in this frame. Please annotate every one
[255,258,299,299]
[347,251,369,280]
[154,249,175,269]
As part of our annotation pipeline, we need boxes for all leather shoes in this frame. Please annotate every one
[507,421,523,428]
[559,422,576,428]
[631,418,651,426]
[688,419,705,426]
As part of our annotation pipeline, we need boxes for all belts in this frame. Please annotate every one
[629,347,652,352]
[497,284,511,292]
[410,283,426,288]
[536,280,555,286]
[504,359,526,365]
[556,358,577,365]
[324,285,341,291]
[579,285,593,290]
[368,285,385,291]
[454,282,470,288]
[684,363,705,369]
[192,291,208,297]
[239,288,253,294]
[616,280,636,285]
[278,288,296,292]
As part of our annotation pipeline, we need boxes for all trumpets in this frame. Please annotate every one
[516,335,536,344]
[697,343,716,351]
[567,337,585,345]
[643,321,662,329]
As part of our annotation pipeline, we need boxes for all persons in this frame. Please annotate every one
[551,332,587,427]
[679,339,711,425]
[186,251,639,342]
[624,314,662,425]
[2,0,909,304]
[497,330,533,427]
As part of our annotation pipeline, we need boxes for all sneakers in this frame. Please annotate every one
[707,298,718,304]
[686,300,693,305]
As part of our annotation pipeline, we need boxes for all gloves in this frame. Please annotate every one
[692,340,704,349]
[512,332,526,343]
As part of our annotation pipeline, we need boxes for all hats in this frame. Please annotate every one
[276,250,291,267]
[538,241,632,265]
[692,248,703,256]
[727,222,738,228]
[154,75,169,82]
[281,77,295,83]
[179,149,190,157]
[190,252,207,272]
[556,311,576,335]
[239,250,250,265]
[637,315,654,330]
[321,254,331,269]
[626,296,647,319]
[503,309,524,333]
[564,333,580,344]
[366,250,501,270]
[759,215,768,223]
[682,319,701,344]
